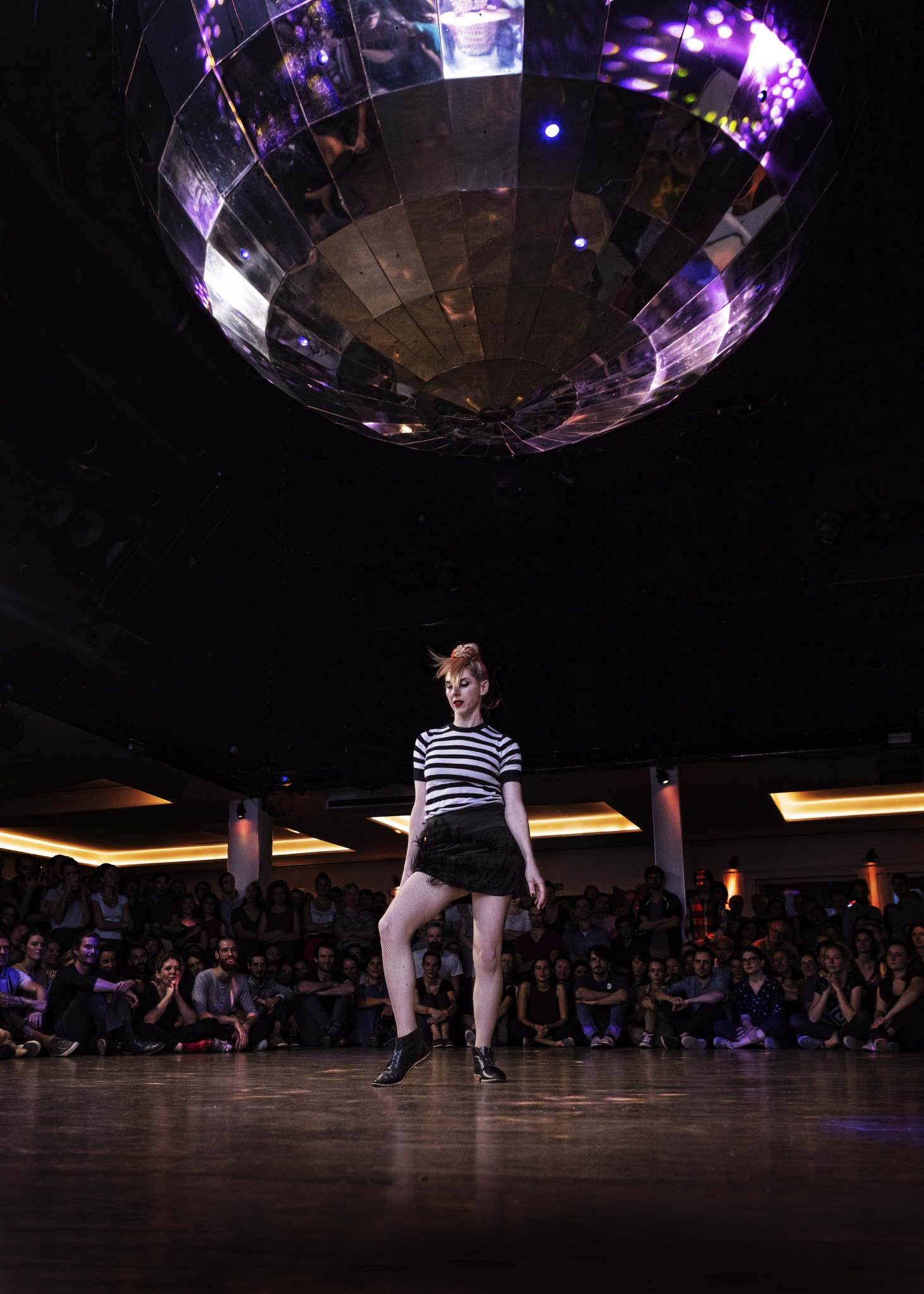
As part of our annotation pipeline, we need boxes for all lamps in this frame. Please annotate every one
[235,803,247,818]
[655,766,671,785]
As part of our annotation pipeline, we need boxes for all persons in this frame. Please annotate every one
[0,843,924,1059]
[372,643,547,1086]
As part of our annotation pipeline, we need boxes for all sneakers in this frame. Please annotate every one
[639,1030,655,1048]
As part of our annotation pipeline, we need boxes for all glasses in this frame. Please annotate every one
[587,892,599,897]
[591,959,606,963]
[771,927,787,936]
[801,961,817,968]
[274,892,286,896]
[264,951,282,957]
[742,956,759,965]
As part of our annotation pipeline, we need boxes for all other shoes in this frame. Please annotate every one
[443,1038,453,1047]
[601,1035,614,1048]
[798,1035,821,1049]
[561,1037,574,1047]
[465,1029,476,1046]
[369,1029,386,1048]
[523,1037,533,1048]
[0,1034,348,1057]
[432,1038,444,1048]
[843,1036,859,1051]
[862,1042,880,1052]
[590,1036,602,1048]
[661,1035,681,1049]
[713,1037,734,1049]
[764,1037,780,1049]
[874,1039,899,1053]
[681,1036,707,1049]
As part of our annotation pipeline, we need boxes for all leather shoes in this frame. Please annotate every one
[473,1046,506,1081]
[373,1040,431,1085]
